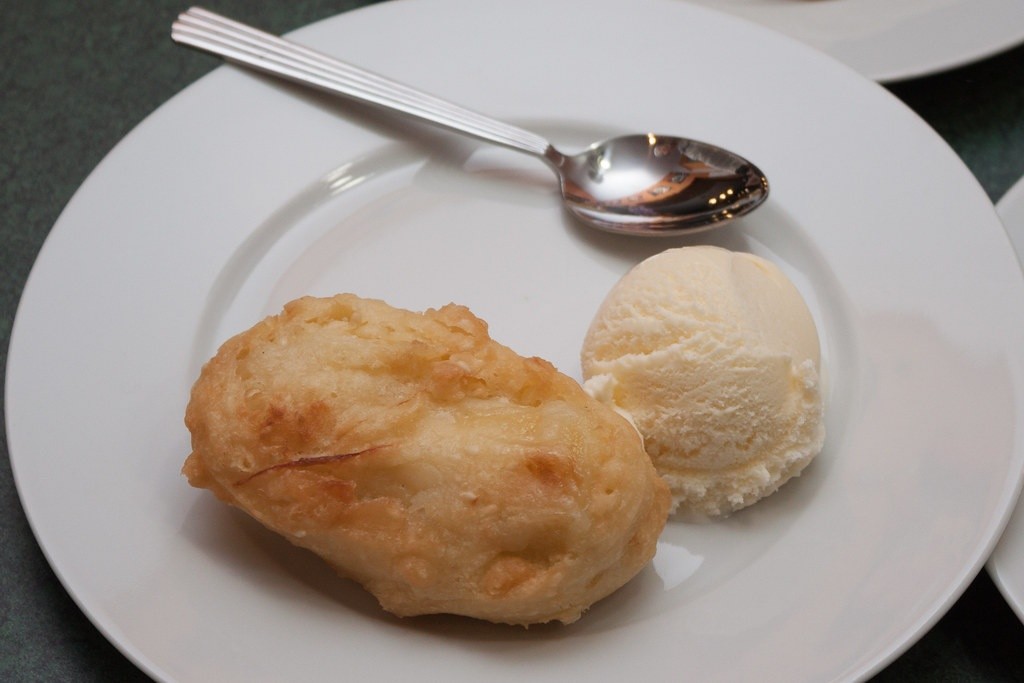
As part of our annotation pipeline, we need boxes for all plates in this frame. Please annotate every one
[4,2,1023,683]
[974,154,1024,635]
[677,0,1022,110]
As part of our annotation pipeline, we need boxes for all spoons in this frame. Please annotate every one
[169,4,772,234]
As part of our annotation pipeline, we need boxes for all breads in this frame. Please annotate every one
[177,292,662,628]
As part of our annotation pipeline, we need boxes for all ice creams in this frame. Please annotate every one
[579,245,825,520]
